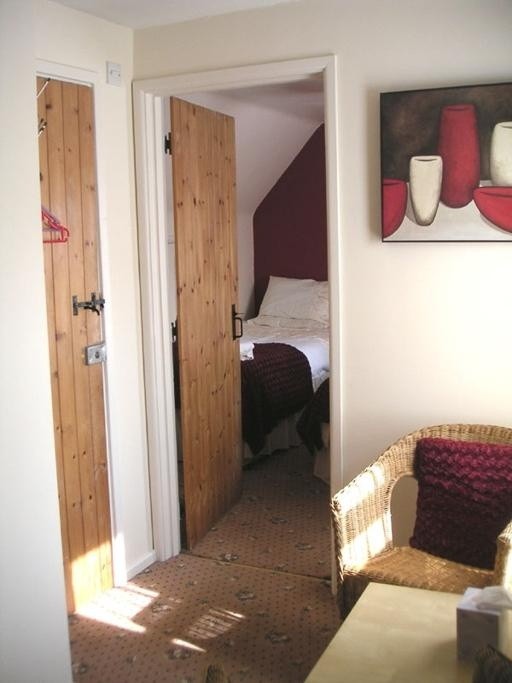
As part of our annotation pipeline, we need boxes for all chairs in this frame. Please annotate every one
[330,425,511,623]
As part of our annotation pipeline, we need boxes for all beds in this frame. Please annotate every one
[239,314,331,467]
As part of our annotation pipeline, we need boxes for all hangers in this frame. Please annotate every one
[42,204,69,244]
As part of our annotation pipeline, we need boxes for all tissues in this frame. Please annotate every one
[452,584,512,661]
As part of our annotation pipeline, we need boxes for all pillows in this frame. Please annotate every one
[408,439,511,570]
[254,274,329,328]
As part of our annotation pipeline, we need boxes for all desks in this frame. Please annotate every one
[305,579,509,683]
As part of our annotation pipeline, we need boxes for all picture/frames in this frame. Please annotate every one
[380,82,512,244]
